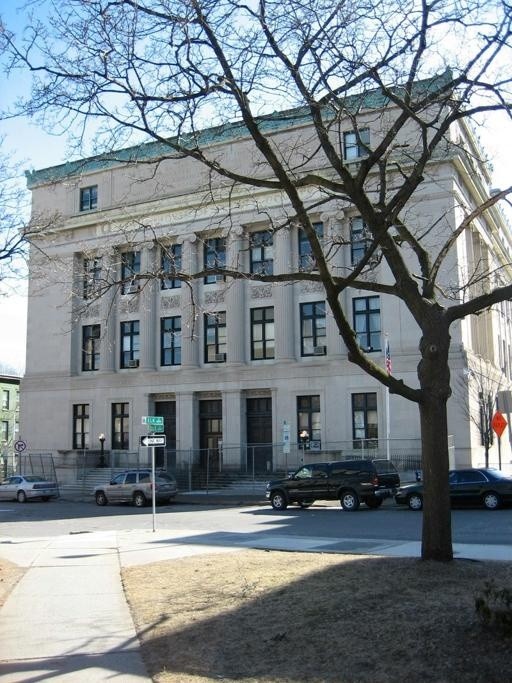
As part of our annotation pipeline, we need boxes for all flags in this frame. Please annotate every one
[384,339,392,375]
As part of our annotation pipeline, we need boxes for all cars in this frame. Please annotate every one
[0,472,60,503]
[394,464,512,512]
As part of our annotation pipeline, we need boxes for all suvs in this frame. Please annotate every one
[90,466,181,507]
[264,456,401,514]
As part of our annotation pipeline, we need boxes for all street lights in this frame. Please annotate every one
[298,429,310,467]
[95,432,110,467]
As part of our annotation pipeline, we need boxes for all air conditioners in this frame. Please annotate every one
[128,284,140,293]
[128,358,139,367]
[313,345,326,355]
[214,274,225,283]
[214,353,226,362]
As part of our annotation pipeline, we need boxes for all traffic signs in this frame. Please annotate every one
[140,415,163,425]
[138,434,166,448]
[145,424,164,432]
